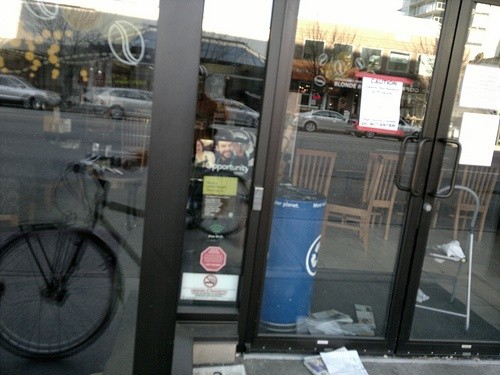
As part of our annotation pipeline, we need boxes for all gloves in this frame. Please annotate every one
[123,155,139,169]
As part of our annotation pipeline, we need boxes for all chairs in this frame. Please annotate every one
[353,150,405,241]
[432,160,500,243]
[322,161,385,254]
[418,185,484,336]
[289,148,337,197]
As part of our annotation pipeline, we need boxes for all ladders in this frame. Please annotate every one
[412,185,480,338]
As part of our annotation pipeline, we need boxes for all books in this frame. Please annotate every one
[307,304,376,338]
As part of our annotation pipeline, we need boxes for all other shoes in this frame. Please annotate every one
[186,207,204,229]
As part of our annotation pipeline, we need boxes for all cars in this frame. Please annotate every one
[207,97,259,130]
[291,108,367,139]
[0,72,61,114]
[82,88,156,122]
[354,112,421,145]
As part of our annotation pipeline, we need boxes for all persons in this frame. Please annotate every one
[195,138,253,173]
[186,65,220,229]
[400,112,411,127]
[311,90,321,106]
[277,121,295,187]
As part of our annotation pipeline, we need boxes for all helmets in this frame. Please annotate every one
[212,126,234,141]
[231,130,249,142]
[198,64,208,76]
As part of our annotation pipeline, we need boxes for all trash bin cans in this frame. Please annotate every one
[260,185,327,333]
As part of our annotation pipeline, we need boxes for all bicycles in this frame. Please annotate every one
[0,149,251,360]
